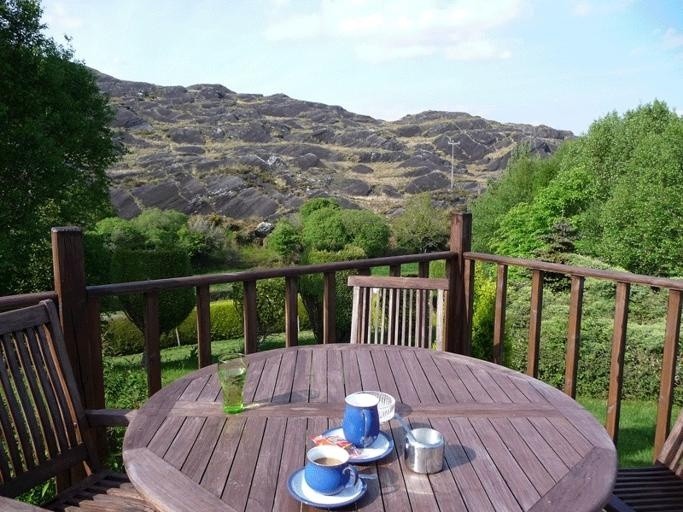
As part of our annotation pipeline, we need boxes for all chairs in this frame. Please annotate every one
[0,298,156,512]
[344,272,451,353]
[599,409,683,512]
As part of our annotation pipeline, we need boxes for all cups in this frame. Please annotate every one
[341,393,379,448]
[216,353,248,413]
[403,428,443,473]
[304,444,357,495]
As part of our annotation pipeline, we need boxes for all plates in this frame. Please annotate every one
[286,466,367,509]
[310,425,394,464]
